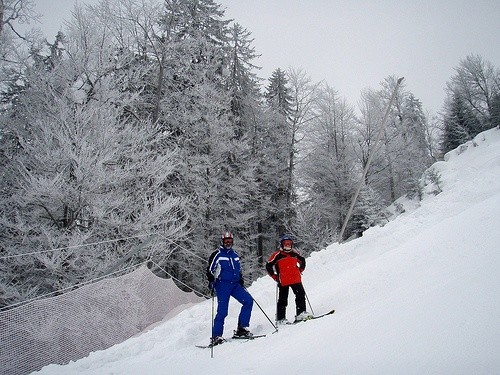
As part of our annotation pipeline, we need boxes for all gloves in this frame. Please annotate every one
[240,279,244,287]
[208,281,215,289]
[272,274,278,281]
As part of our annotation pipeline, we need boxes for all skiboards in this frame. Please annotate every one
[195,334,267,348]
[286,310,335,325]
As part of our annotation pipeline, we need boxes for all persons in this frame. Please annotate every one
[205,232,253,345]
[266,236,312,324]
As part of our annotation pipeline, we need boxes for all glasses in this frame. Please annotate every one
[223,239,233,244]
[283,241,292,247]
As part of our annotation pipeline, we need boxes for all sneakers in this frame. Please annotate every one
[295,311,313,321]
[276,319,290,326]
[236,328,250,337]
[210,336,225,346]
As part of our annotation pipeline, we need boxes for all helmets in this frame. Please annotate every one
[279,236,293,252]
[221,232,233,249]
